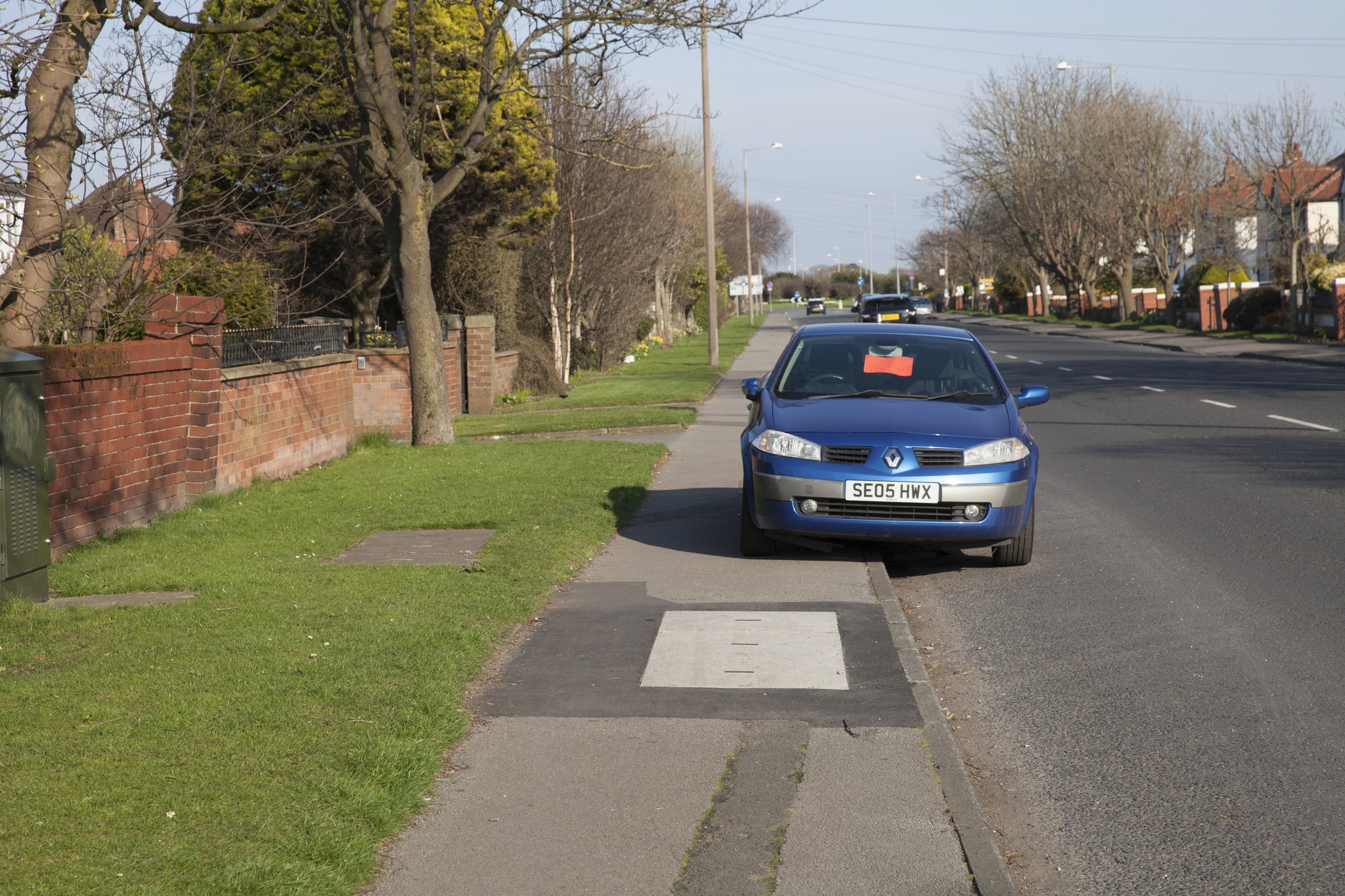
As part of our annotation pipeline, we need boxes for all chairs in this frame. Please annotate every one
[810,343,848,384]
[919,343,953,392]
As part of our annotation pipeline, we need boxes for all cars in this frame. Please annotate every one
[804,298,826,316]
[909,296,933,318]
[851,294,920,325]
[737,322,1052,567]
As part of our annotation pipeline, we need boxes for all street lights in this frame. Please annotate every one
[834,246,840,273]
[1054,62,1126,323]
[812,268,821,280]
[914,174,947,310]
[868,192,901,294]
[756,197,782,314]
[742,141,784,326]
[858,260,863,293]
[788,258,793,273]
